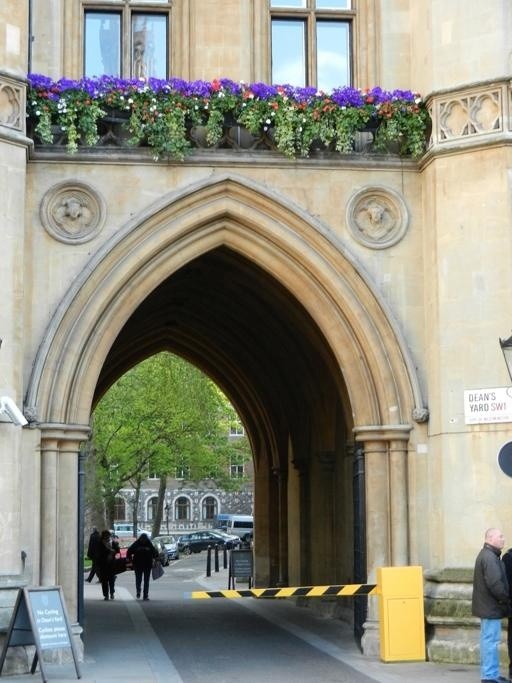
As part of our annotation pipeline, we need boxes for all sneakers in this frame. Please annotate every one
[103,592,115,600]
[136,591,150,601]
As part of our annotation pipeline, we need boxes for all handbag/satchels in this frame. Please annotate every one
[106,550,128,575]
[151,559,165,579]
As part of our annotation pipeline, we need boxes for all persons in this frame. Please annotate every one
[471,527,512,683]
[126,532,158,600]
[83,524,103,583]
[501,547,512,679]
[94,530,120,600]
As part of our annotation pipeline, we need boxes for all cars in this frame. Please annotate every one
[114,525,151,539]
[214,514,253,542]
[152,536,179,567]
[111,538,136,569]
[178,530,240,555]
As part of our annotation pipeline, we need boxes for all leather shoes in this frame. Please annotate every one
[480,675,512,683]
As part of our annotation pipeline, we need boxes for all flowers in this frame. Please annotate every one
[24,71,429,161]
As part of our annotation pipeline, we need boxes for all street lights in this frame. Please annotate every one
[165,501,171,536]
